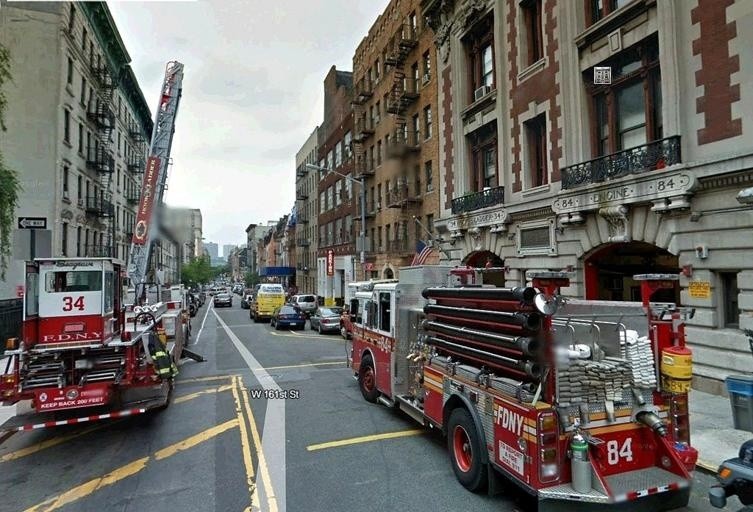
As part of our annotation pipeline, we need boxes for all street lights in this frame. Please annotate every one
[178,237,206,284]
[305,163,365,283]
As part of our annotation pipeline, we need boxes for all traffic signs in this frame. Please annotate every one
[17,216,46,229]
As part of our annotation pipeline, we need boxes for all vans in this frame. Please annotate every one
[249,283,286,323]
[286,293,318,316]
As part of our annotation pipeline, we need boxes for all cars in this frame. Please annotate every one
[309,305,344,335]
[190,273,254,317]
[270,305,306,330]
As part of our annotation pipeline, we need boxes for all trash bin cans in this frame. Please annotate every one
[726,375,753,433]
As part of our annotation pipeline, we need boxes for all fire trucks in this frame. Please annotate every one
[347,262,697,507]
[1,256,191,434]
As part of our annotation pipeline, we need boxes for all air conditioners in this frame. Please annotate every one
[474,86,490,100]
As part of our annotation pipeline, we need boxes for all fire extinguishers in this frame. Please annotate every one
[570,424,593,494]
